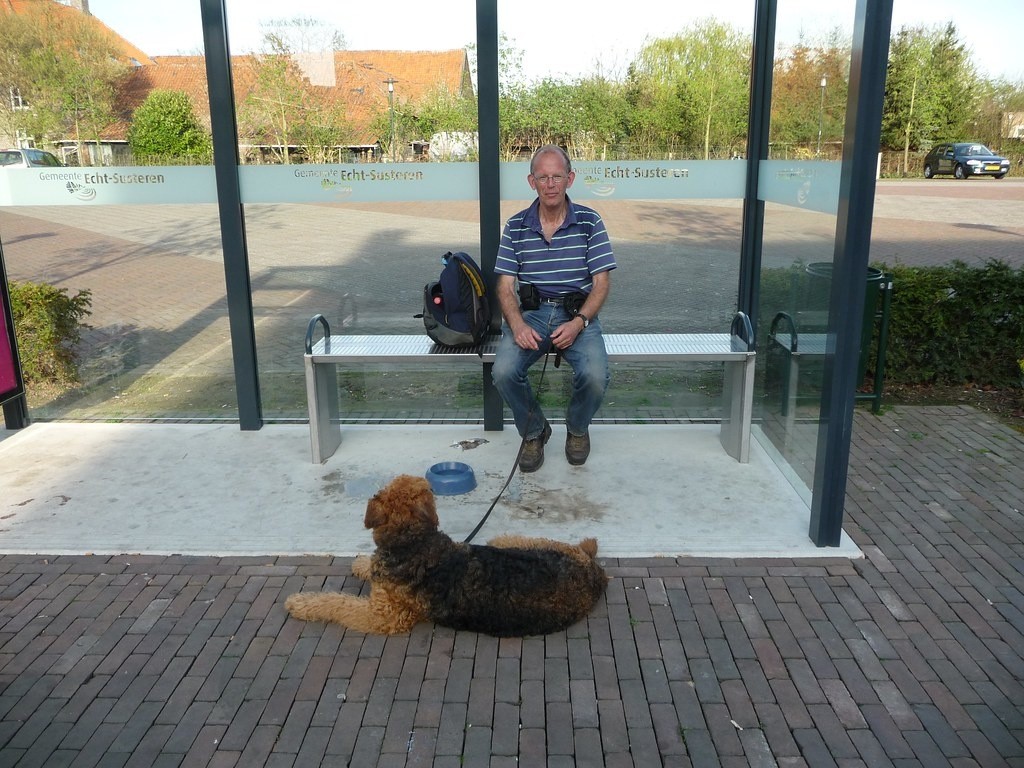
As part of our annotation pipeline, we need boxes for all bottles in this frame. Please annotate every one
[432,294,443,308]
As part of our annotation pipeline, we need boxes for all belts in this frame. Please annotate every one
[541,297,565,304]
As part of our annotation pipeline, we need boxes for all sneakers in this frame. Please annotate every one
[565,428,590,466]
[519,418,552,472]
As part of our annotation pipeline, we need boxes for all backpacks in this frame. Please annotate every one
[413,251,491,359]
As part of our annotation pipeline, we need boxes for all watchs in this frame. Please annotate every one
[574,313,588,328]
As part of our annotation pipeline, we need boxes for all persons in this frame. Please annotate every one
[491,145,619,472]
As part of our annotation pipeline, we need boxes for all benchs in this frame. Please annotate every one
[768,312,837,456]
[300,311,759,464]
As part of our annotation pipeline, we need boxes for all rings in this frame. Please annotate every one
[521,341,525,344]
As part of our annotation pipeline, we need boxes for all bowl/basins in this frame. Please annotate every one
[425,461,477,495]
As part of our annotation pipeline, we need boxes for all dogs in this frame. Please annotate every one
[283,473,608,637]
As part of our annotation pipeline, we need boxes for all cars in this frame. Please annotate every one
[923,142,1010,179]
[0,148,79,179]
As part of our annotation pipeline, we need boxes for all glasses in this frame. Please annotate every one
[533,173,569,185]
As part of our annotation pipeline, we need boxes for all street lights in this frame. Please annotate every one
[384,77,398,160]
[818,76,827,148]
